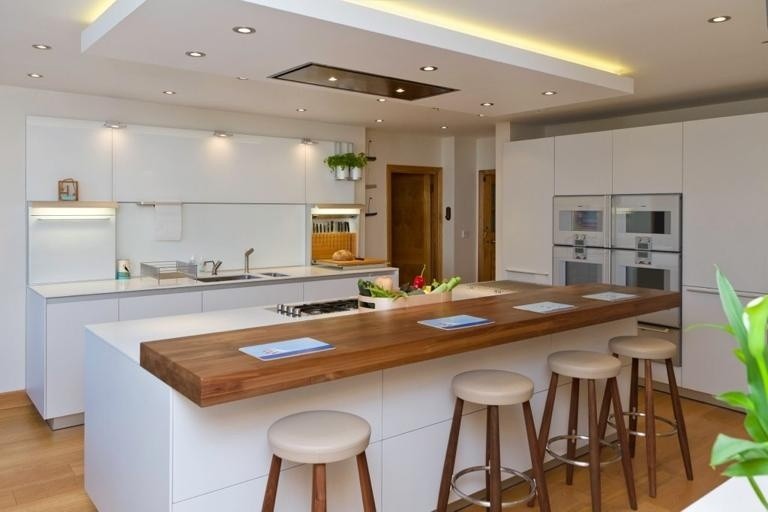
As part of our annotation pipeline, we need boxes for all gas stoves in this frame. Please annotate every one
[272,296,376,318]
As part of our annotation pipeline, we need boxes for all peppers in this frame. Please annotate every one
[413,265,426,289]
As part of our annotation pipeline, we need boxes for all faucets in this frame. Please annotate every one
[204,260,222,274]
[244,248,254,273]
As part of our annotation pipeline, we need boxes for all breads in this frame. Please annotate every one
[332,249,352,261]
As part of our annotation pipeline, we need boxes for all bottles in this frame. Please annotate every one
[367,139,376,157]
[367,197,377,213]
[117,259,131,280]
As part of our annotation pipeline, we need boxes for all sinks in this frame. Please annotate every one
[262,272,289,278]
[196,275,264,283]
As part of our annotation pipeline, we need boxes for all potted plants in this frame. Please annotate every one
[323,152,368,181]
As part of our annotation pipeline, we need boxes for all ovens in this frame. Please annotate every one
[552,192,682,367]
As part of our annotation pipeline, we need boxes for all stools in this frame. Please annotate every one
[436,370,551,512]
[538,350,638,512]
[599,335,692,499]
[260,409,380,511]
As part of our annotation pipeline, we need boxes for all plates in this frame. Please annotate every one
[359,292,452,308]
